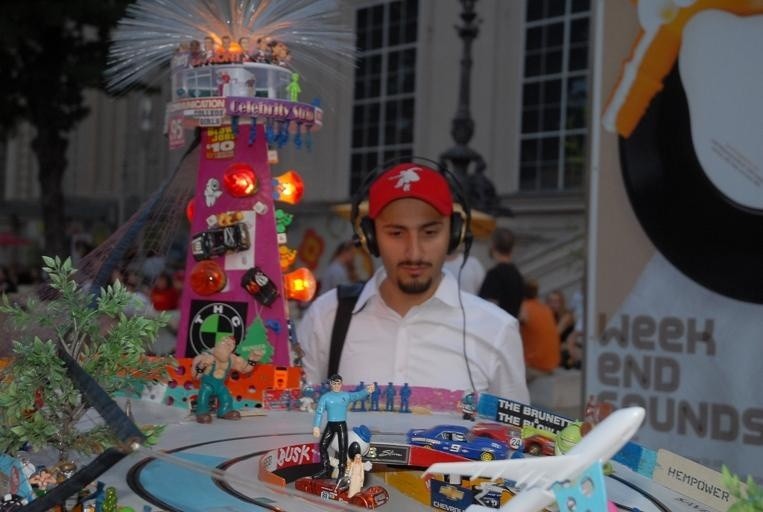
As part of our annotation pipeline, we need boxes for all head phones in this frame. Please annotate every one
[352,156,473,258]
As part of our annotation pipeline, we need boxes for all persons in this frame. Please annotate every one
[191,336,264,425]
[319,238,355,294]
[265,116,276,149]
[247,116,258,145]
[475,226,526,318]
[287,73,303,101]
[306,124,313,150]
[383,381,397,411]
[276,119,290,148]
[351,381,367,411]
[516,279,560,386]
[547,291,576,370]
[399,382,410,412]
[368,381,381,412]
[289,162,530,413]
[171,34,294,69]
[461,393,475,422]
[348,454,364,499]
[30,469,57,497]
[316,382,329,400]
[296,384,315,412]
[294,121,304,149]
[313,374,375,485]
[231,114,239,135]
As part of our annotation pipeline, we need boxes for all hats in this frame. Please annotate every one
[367,162,453,220]
